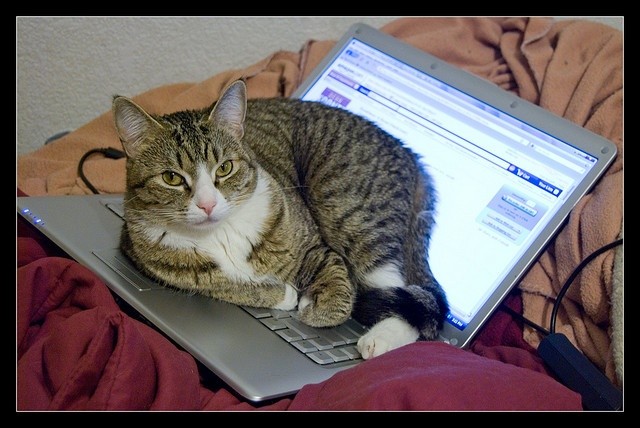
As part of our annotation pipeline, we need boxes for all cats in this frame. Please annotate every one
[112,74,451,360]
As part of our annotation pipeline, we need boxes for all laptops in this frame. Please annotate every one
[16,22,619,406]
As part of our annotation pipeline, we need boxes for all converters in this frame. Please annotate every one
[535,332,622,411]
